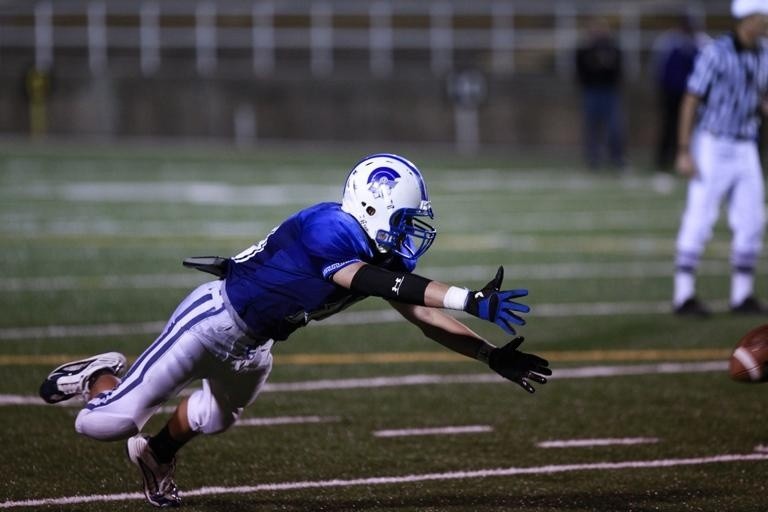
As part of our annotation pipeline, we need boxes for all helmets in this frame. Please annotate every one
[338,151,434,256]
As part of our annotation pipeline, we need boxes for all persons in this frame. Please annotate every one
[671,0,768,313]
[576,28,623,168]
[41,153,552,508]
[651,17,710,169]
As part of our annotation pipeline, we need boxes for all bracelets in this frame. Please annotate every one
[475,344,495,363]
[441,286,468,311]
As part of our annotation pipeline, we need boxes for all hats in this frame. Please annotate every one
[728,0,768,20]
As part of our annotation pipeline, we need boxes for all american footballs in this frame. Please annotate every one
[729,324,767,383]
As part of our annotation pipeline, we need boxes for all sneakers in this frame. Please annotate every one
[674,294,768,317]
[125,434,184,507]
[38,350,127,404]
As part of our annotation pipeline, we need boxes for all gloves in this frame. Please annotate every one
[462,263,531,335]
[487,336,553,394]
